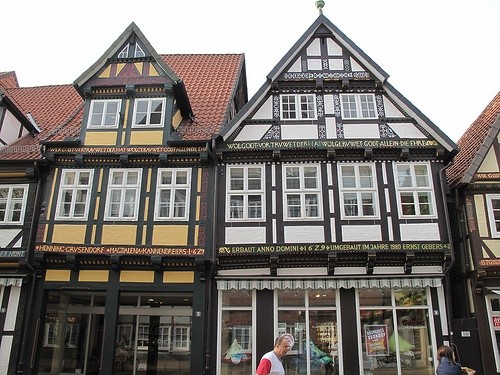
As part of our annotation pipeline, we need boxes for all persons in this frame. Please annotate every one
[117,335,129,352]
[255,336,290,375]
[435,346,477,375]
